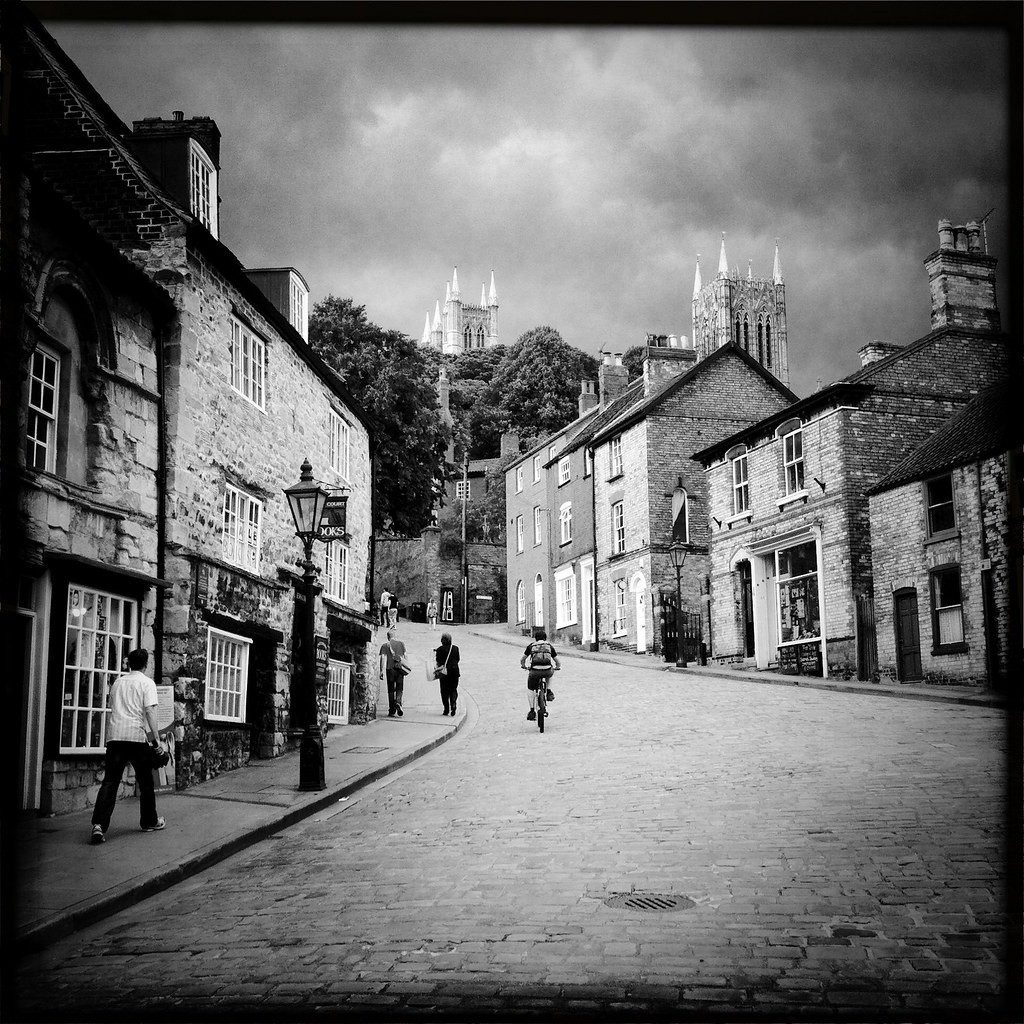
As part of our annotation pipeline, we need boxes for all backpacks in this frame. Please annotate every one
[529,642,552,665]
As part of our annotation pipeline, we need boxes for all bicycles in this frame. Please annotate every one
[520,664,561,734]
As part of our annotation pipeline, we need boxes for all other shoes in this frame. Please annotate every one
[547,692,554,701]
[442,710,449,715]
[526,711,536,720]
[395,702,404,717]
[450,705,457,717]
[388,713,395,717]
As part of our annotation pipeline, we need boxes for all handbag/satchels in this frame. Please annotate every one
[392,654,411,676]
[433,666,447,680]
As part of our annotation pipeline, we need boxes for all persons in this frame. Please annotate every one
[90,649,167,843]
[427,598,438,630]
[436,633,461,717]
[521,630,561,721]
[388,591,399,630]
[379,587,391,627]
[379,630,407,717]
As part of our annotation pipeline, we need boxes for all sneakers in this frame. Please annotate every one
[140,815,167,832]
[90,823,106,843]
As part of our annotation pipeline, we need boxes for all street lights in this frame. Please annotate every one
[281,458,330,791]
[669,534,694,668]
[460,449,471,624]
